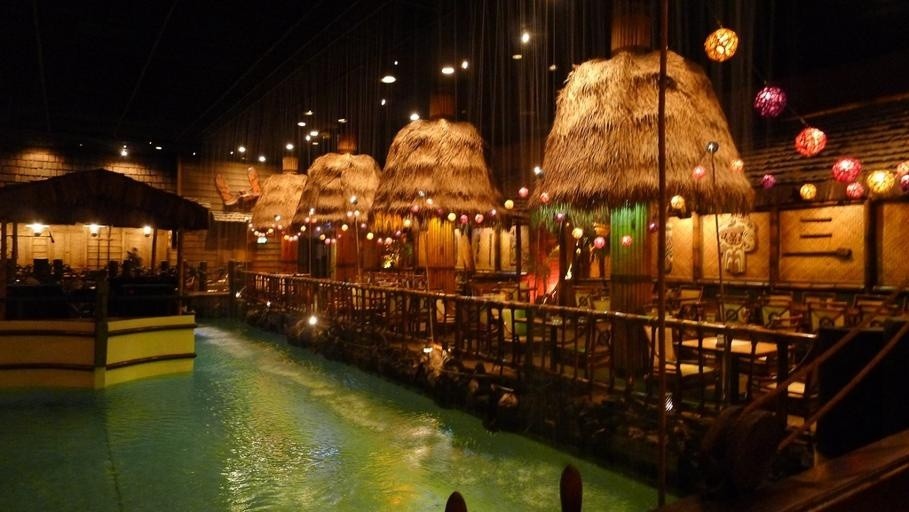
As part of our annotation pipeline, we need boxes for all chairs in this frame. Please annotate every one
[251,266,909,471]
[1,256,231,315]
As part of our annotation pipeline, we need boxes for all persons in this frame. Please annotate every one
[126,247,143,268]
